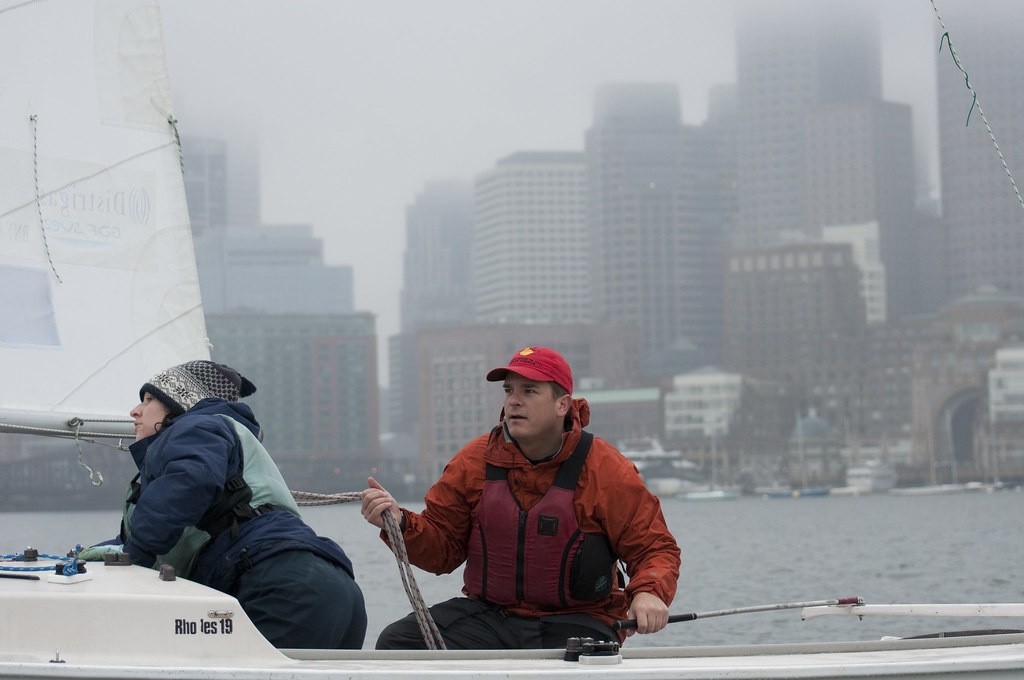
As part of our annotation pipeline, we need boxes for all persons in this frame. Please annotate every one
[76,359,369,649]
[356,345,682,650]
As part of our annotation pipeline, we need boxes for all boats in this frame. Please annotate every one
[0,0,1022,680]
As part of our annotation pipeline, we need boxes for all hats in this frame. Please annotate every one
[486,345,574,396]
[139,360,257,414]
